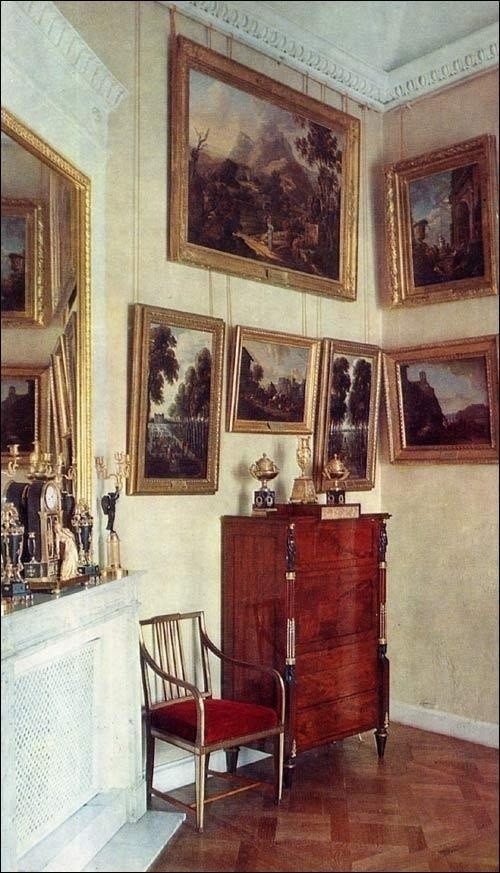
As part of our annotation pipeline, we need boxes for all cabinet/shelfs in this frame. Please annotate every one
[220,513,394,788]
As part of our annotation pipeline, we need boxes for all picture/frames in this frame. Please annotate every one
[168,34,360,302]
[313,338,383,494]
[224,325,321,435]
[0,368,50,462]
[0,201,44,329]
[383,335,499,465]
[383,133,499,307]
[126,302,226,497]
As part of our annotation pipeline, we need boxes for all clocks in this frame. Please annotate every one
[26,479,63,591]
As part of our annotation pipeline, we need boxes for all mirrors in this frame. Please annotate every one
[0,106,99,600]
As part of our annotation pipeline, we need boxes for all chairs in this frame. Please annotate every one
[138,610,285,833]
